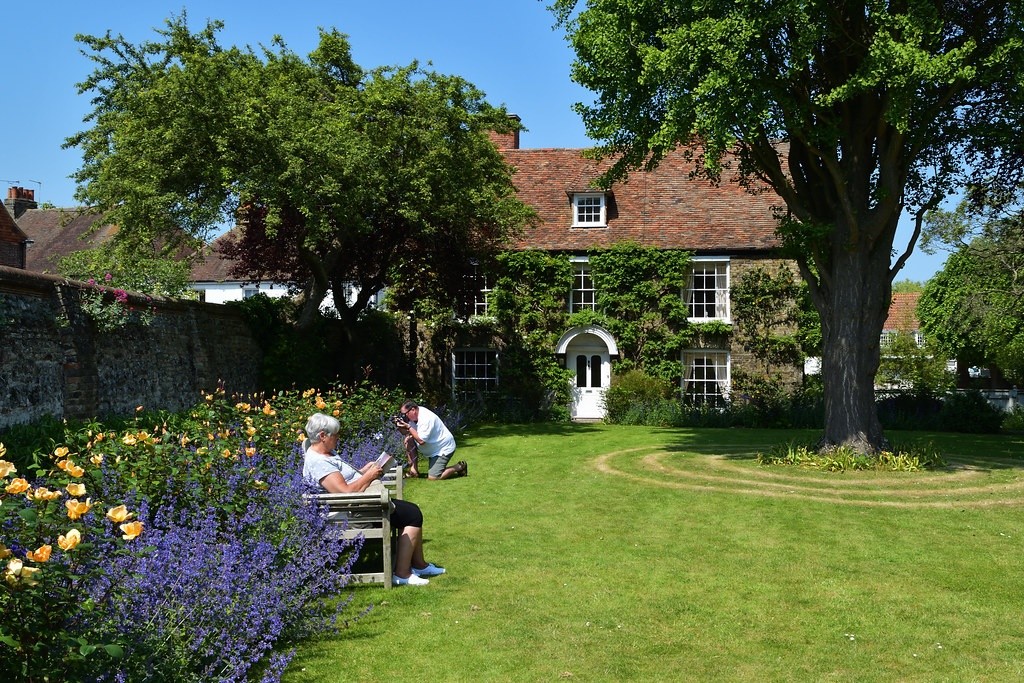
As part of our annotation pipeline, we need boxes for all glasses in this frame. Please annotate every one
[326,432,340,439]
[401,409,411,416]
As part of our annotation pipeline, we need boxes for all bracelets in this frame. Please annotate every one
[407,426,412,430]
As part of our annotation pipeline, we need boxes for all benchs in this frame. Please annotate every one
[300,437,403,590]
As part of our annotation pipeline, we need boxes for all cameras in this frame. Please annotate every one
[396,413,409,424]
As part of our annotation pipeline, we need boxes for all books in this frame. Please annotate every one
[370,451,398,481]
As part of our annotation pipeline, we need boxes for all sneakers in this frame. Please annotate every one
[411,563,445,575]
[392,574,429,585]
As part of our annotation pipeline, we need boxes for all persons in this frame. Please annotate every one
[394,398,468,480]
[300,413,447,586]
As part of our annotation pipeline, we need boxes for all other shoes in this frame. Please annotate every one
[404,470,420,479]
[457,461,468,477]
